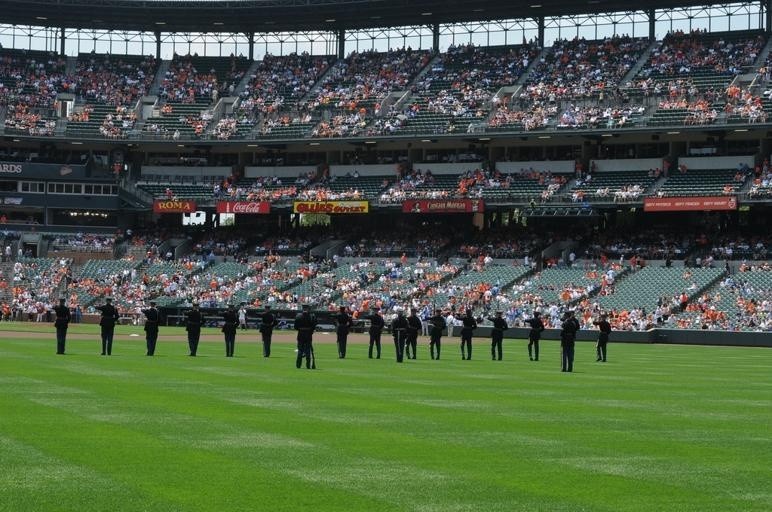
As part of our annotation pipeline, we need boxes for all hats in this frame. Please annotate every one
[371,307,381,312]
[410,308,418,313]
[339,305,345,308]
[492,310,506,314]
[58,298,67,303]
[150,301,157,305]
[227,304,235,307]
[396,308,405,315]
[301,303,312,309]
[192,302,201,307]
[435,310,442,313]
[106,296,114,302]
[264,304,270,308]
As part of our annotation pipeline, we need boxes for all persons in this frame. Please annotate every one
[258,305,276,357]
[559,311,580,372]
[486,311,509,360]
[140,300,160,356]
[429,309,446,359]
[362,306,385,359]
[333,306,353,358]
[522,312,546,361]
[220,304,240,356]
[182,302,202,356]
[593,313,612,362]
[456,309,478,360]
[50,297,71,354]
[293,304,315,368]
[392,308,410,362]
[404,308,422,360]
[93,297,119,355]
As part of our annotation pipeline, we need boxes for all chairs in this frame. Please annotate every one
[1,229,771,334]
[6,30,771,210]
